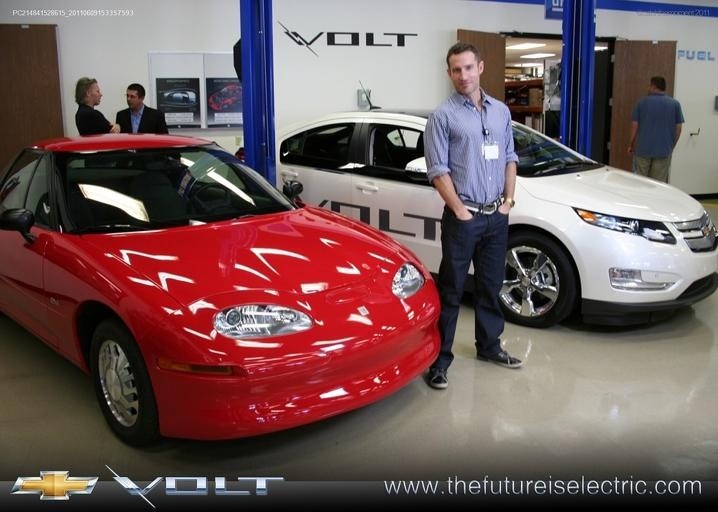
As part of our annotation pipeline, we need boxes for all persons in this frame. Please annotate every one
[74,77,120,136]
[423,41,523,389]
[117,84,167,134]
[628,77,684,182]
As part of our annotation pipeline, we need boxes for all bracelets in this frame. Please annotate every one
[504,197,515,207]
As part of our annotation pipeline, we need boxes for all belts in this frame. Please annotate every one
[463,194,505,215]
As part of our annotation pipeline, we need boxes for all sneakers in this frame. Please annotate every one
[477,351,523,368]
[429,368,448,388]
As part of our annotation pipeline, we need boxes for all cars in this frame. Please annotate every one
[208,84,242,110]
[163,90,196,105]
